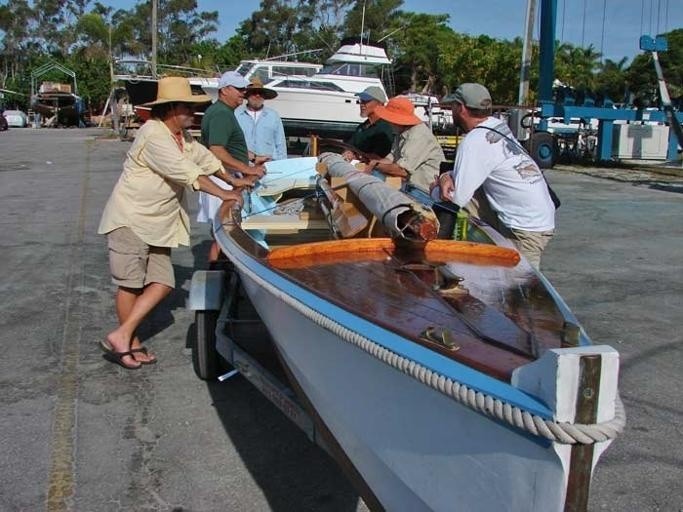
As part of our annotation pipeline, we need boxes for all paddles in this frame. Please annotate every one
[318,178,369,238]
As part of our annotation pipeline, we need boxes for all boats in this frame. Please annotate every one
[111,0,453,150]
[189,156,626,512]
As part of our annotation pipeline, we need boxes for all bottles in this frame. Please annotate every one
[450,208,469,240]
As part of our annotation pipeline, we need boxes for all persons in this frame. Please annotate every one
[423,81,561,272]
[195,68,273,268]
[337,85,394,166]
[356,96,446,193]
[91,75,257,374]
[236,71,288,165]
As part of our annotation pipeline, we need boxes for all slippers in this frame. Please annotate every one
[99,340,155,369]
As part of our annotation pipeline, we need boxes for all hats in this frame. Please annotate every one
[217,72,250,87]
[247,75,278,100]
[144,77,212,107]
[440,83,493,111]
[356,86,386,102]
[375,97,422,126]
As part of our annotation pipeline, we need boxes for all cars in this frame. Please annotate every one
[0,113,8,131]
[2,110,26,129]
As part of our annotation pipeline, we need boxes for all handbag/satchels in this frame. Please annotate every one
[547,186,560,209]
